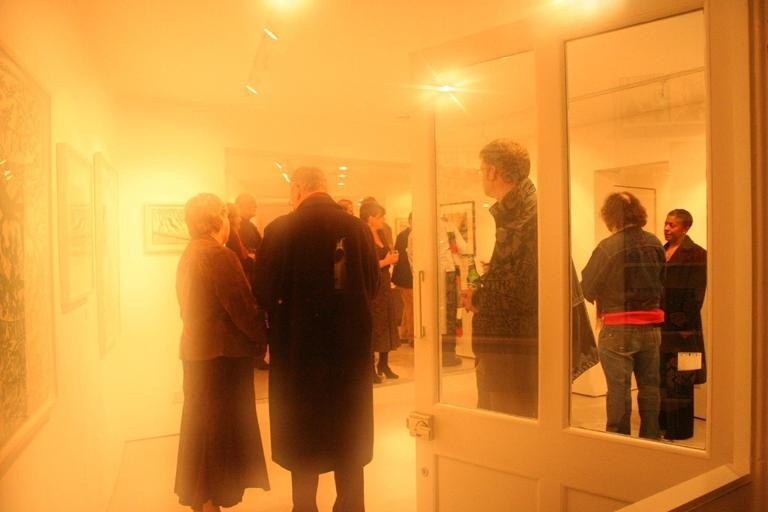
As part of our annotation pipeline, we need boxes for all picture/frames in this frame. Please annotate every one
[144,204,192,255]
[395,217,409,237]
[91,152,122,356]
[439,201,475,257]
[56,144,89,312]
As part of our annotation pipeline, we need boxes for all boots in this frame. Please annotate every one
[377,363,397,378]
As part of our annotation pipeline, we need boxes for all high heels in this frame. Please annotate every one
[372,370,379,381]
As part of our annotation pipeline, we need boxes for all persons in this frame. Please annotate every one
[457,136,601,441]
[659,208,710,441]
[581,192,667,442]
[174,163,473,512]
